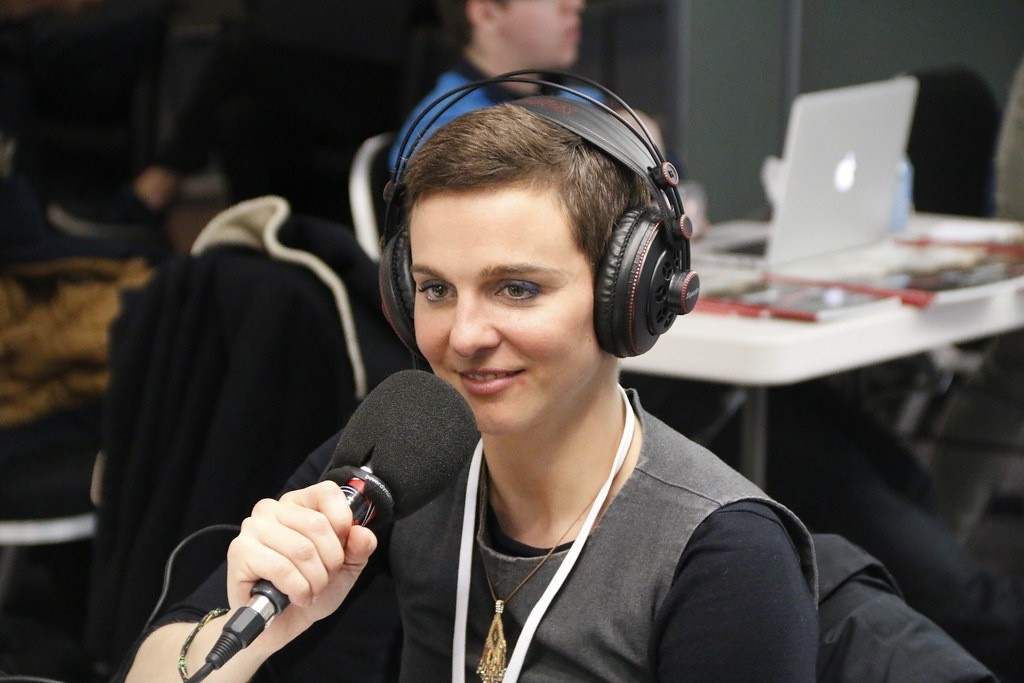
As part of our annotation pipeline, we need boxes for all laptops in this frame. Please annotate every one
[685,75,924,271]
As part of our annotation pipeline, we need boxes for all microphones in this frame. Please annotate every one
[183,368,483,683]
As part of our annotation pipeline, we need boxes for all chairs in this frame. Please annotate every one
[349,133,397,265]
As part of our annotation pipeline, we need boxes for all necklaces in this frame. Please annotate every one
[480,489,601,683]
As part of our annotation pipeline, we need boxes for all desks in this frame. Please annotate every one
[612,212,1024,500]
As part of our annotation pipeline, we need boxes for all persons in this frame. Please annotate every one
[122,95,821,683]
[389,0,1024,683]
[135,72,426,394]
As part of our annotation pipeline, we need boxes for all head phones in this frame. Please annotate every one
[377,65,702,358]
